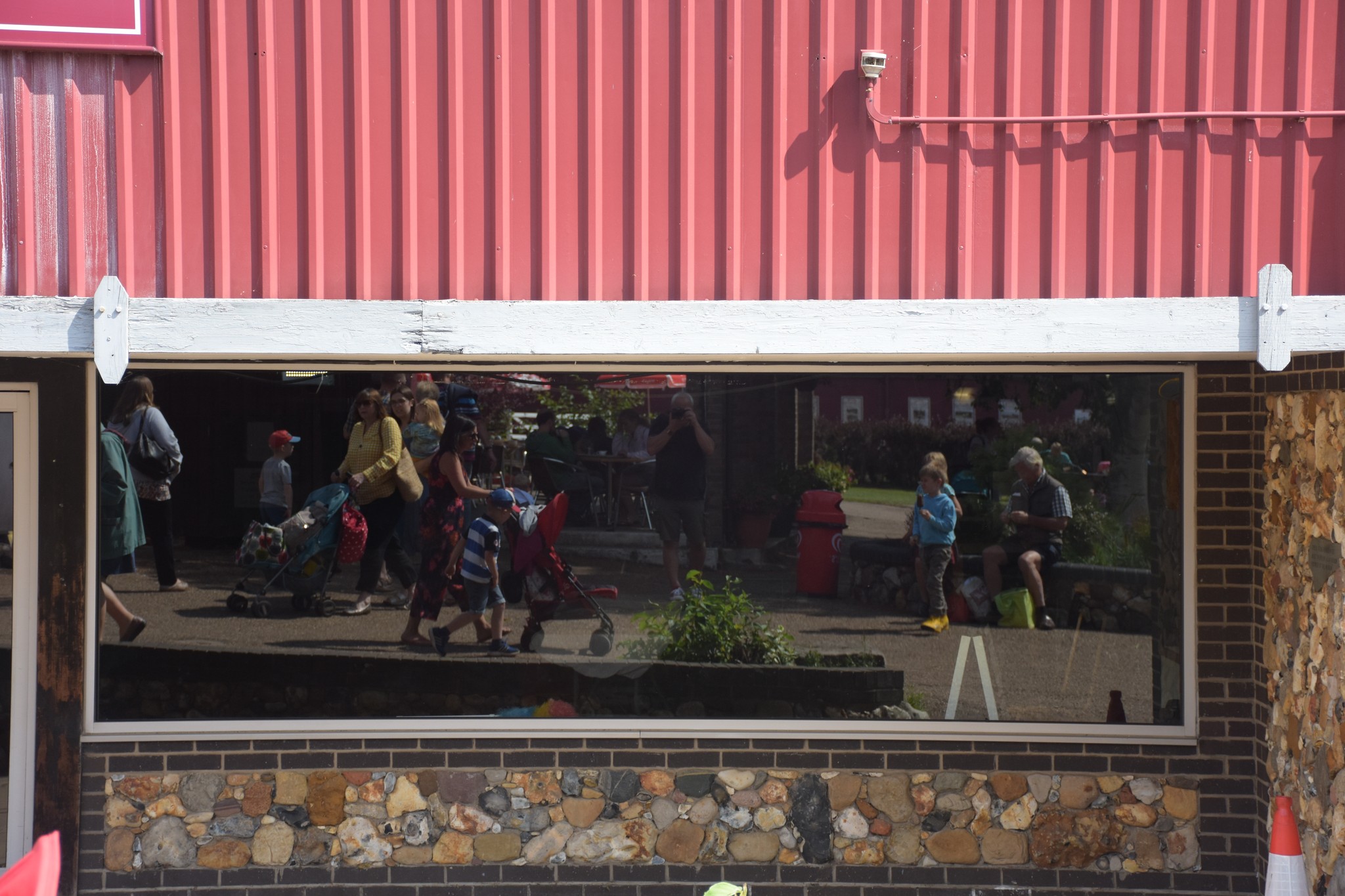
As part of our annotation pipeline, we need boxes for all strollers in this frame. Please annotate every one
[489,472,618,655]
[226,470,359,609]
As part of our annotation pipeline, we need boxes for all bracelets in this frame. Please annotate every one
[335,470,340,476]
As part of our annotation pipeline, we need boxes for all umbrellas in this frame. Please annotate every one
[594,374,686,425]
[451,373,551,395]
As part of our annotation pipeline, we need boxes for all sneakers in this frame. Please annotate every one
[487,639,520,656]
[921,613,949,633]
[429,626,450,657]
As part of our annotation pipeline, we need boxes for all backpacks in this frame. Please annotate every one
[947,587,970,622]
[277,501,332,550]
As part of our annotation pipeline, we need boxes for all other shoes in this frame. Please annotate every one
[477,626,512,644]
[670,586,688,602]
[399,634,431,647]
[689,586,704,599]
[383,591,415,606]
[1039,614,1055,628]
[119,615,146,643]
[159,579,190,590]
[340,599,372,616]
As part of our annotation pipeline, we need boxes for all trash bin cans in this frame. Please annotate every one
[795,490,850,595]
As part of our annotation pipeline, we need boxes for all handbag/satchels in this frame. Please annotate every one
[379,417,424,503]
[127,406,170,478]
[960,574,992,620]
[337,499,368,565]
[995,587,1036,629]
[234,518,292,568]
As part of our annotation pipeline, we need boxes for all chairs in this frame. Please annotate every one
[472,437,656,530]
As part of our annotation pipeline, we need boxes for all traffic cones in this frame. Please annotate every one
[1264,796,1308,896]
[1106,689,1127,724]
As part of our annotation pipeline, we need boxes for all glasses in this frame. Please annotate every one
[354,399,373,409]
[464,430,479,440]
[388,398,406,406]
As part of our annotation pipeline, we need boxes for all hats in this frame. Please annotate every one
[488,489,514,510]
[268,430,301,449]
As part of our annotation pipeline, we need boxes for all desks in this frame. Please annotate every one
[576,453,644,530]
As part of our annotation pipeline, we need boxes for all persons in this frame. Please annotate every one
[399,416,510,647]
[427,488,521,658]
[646,392,715,601]
[99,421,148,644]
[259,430,301,527]
[899,452,963,632]
[108,376,190,592]
[525,408,657,526]
[981,446,1072,629]
[331,372,479,616]
[965,421,1074,467]
